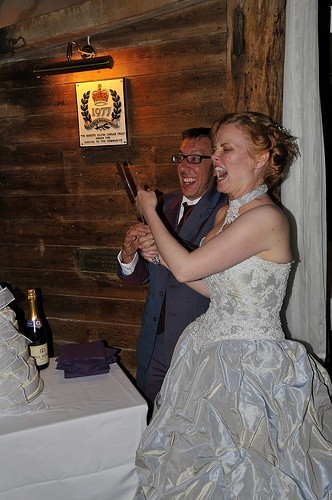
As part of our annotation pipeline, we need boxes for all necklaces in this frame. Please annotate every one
[217,183,268,233]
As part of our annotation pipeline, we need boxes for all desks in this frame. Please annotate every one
[0,357,148,500]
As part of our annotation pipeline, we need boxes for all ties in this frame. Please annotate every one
[177,202,197,233]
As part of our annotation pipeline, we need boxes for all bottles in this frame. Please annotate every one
[24,289,49,370]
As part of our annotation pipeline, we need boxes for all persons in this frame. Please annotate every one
[116,127,229,427]
[133,112,332,500]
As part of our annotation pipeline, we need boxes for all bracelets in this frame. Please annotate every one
[152,254,160,265]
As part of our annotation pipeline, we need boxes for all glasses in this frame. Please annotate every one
[172,153,212,164]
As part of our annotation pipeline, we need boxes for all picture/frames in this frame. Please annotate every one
[74,77,129,149]
